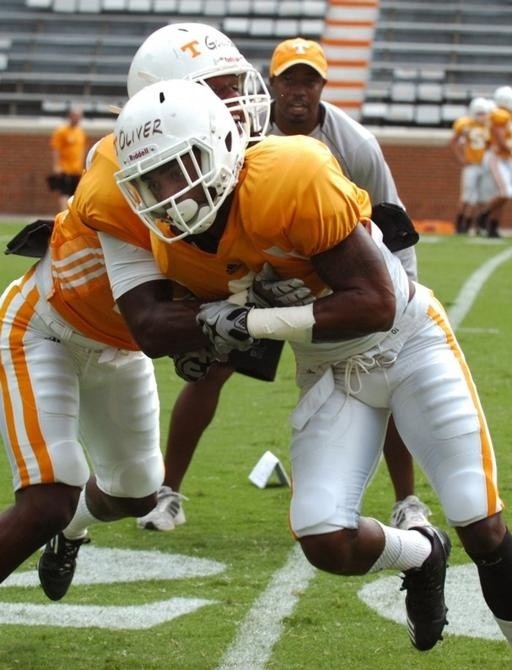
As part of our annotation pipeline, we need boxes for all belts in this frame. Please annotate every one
[49,320,110,352]
[286,280,431,432]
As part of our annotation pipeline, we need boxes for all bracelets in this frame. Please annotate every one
[247,303,316,348]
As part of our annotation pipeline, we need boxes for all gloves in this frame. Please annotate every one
[246,261,319,309]
[195,301,252,355]
[172,346,228,385]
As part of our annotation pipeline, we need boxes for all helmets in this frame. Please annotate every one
[469,98,489,123]
[112,79,246,244]
[126,22,273,143]
[494,87,512,110]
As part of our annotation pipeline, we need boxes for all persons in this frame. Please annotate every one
[46,104,91,213]
[1,23,15,69]
[133,33,437,534]
[0,17,318,602]
[472,84,511,239]
[111,72,510,654]
[445,97,493,235]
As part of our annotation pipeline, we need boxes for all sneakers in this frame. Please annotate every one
[389,499,433,531]
[135,485,186,531]
[397,522,451,652]
[38,529,89,600]
[476,226,490,238]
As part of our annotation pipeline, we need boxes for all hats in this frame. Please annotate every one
[269,37,329,82]
